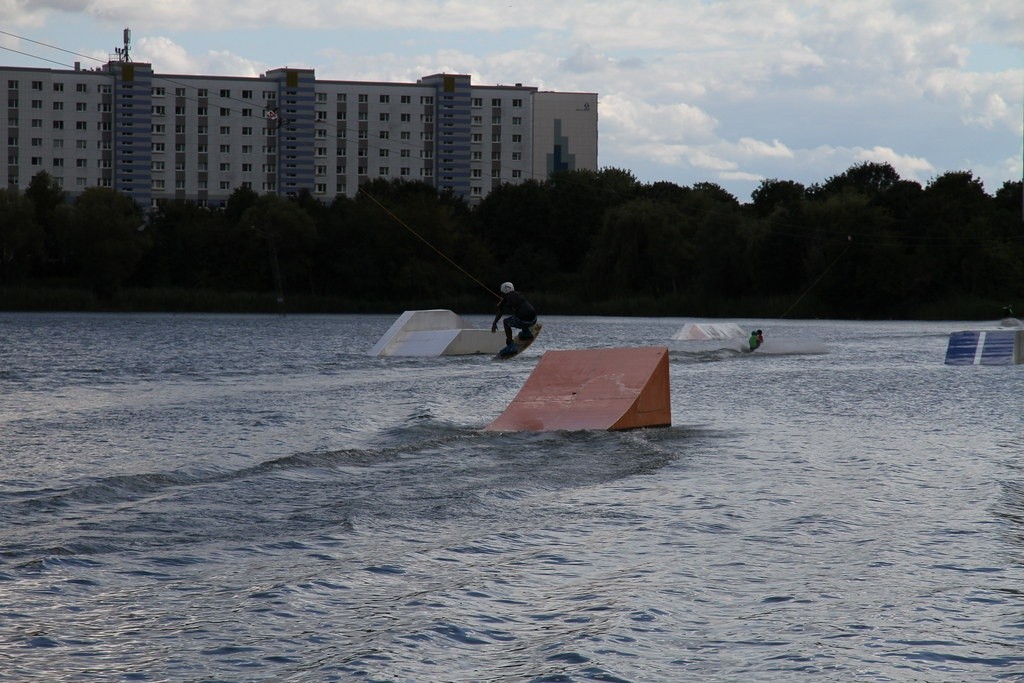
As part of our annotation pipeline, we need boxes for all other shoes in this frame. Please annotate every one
[506,340,517,353]
[518,331,534,340]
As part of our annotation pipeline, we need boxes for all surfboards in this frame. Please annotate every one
[493,323,543,361]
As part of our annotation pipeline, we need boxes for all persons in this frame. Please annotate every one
[748,331,762,351]
[491,282,537,355]
[756,329,763,347]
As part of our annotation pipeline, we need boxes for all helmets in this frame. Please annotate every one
[500,281,515,293]
